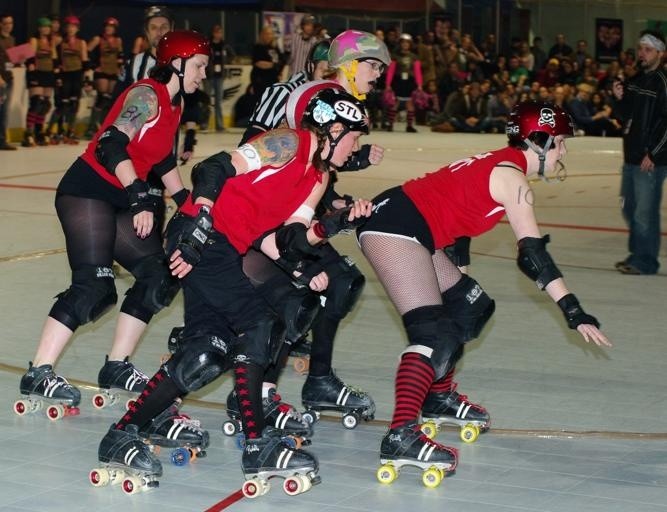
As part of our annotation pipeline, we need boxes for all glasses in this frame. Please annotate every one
[363,58,387,75]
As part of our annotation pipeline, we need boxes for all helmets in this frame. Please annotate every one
[36,13,120,31]
[283,77,348,130]
[140,4,173,30]
[303,38,332,79]
[326,27,393,70]
[398,32,415,43]
[152,26,215,67]
[300,14,319,29]
[302,87,373,136]
[507,99,577,144]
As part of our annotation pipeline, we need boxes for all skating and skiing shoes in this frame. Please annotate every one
[239,426,324,499]
[296,370,376,431]
[11,357,82,421]
[91,354,150,414]
[376,423,462,488]
[421,389,493,446]
[86,421,164,495]
[139,396,211,469]
[223,340,315,452]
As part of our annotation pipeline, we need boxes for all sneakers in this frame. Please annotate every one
[387,126,392,132]
[615,254,661,275]
[407,127,417,132]
[17,124,96,149]
